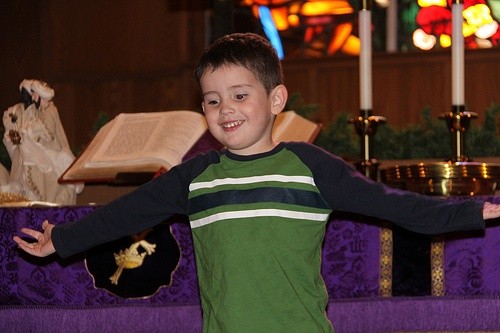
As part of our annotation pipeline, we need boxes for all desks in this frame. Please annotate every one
[72,158,499,208]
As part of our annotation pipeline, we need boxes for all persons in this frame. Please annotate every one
[12,34,500,332]
[2,79,81,207]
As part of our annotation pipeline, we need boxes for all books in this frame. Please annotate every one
[56,111,326,184]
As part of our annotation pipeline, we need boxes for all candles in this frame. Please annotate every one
[358,0,373,110]
[450,0,466,112]
[385,1,399,53]
